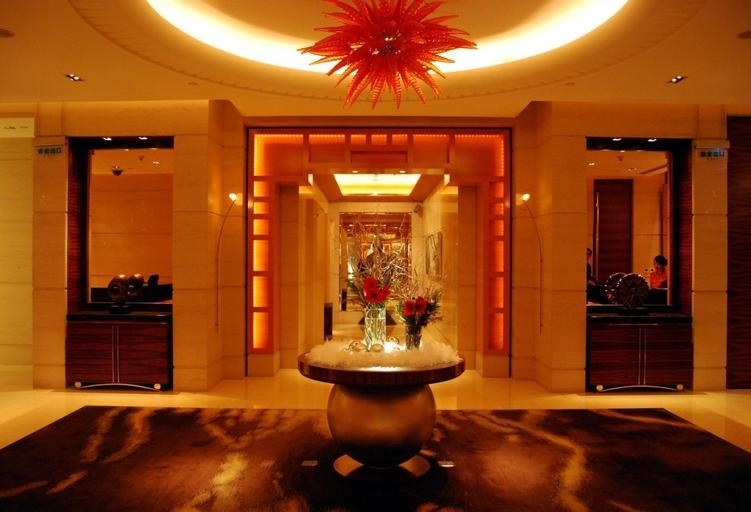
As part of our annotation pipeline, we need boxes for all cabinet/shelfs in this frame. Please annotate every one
[64,318,170,390]
[586,312,693,394]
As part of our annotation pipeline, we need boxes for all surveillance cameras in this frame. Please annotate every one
[110,166,124,177]
[414,204,421,213]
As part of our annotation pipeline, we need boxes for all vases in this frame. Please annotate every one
[405,333,423,349]
[363,308,386,348]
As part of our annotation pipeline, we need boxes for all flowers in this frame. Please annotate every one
[396,283,444,330]
[344,266,392,308]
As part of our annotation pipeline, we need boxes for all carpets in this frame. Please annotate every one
[1,408,751,512]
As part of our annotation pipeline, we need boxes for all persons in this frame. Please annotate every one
[650,254,669,288]
[587,248,601,286]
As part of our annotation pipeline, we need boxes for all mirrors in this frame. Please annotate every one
[585,148,676,309]
[78,142,173,309]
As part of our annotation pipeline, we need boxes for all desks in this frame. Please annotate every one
[297,341,465,469]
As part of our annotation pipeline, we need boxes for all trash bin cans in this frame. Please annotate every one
[324,302,332,341]
[341,288,347,311]
